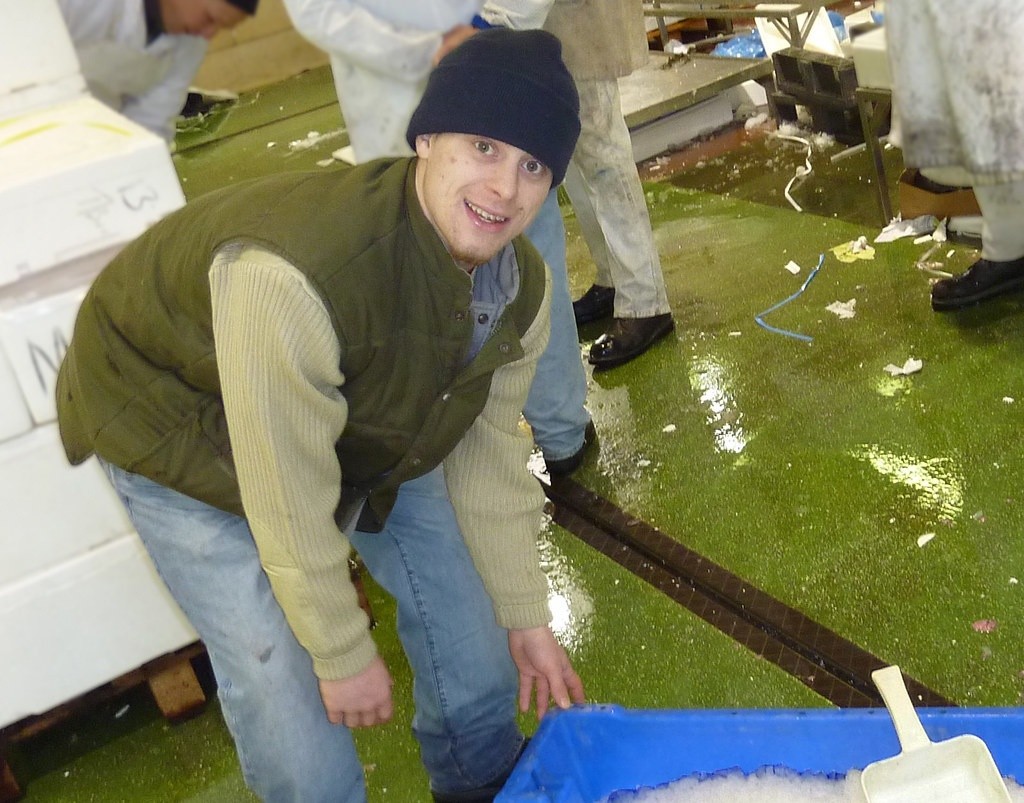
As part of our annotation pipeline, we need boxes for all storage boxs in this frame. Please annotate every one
[753,4,893,90]
[895,167,982,220]
[489,697,1024,803]
[0,0,204,731]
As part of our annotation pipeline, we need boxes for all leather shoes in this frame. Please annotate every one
[589,313,674,364]
[930,253,1024,307]
[571,286,615,325]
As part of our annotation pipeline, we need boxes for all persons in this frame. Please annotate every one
[54,29,584,803]
[882,0,1024,310]
[58,0,674,365]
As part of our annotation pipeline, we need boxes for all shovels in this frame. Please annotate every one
[861,663,1016,803]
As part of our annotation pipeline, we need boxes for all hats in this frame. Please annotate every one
[407,29,583,190]
